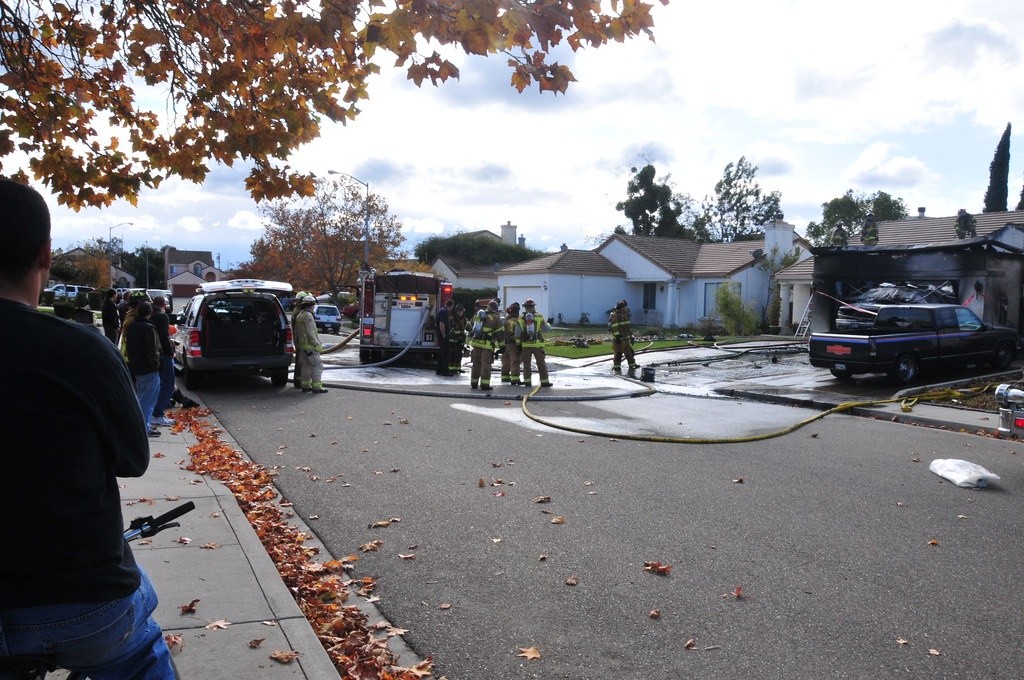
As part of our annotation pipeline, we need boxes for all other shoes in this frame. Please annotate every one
[525,383,531,386]
[480,384,493,390]
[630,364,640,369]
[615,367,621,371]
[502,379,510,382]
[472,384,478,388]
[167,400,176,408]
[541,382,553,387]
[294,381,328,393]
[148,431,161,437]
[511,380,525,385]
[442,368,465,376]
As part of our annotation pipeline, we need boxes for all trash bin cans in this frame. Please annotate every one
[70,308,97,326]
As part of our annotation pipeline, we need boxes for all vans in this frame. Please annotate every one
[43,284,95,302]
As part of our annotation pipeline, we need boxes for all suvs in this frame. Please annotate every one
[314,304,342,333]
[165,277,296,387]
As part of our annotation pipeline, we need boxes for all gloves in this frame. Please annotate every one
[516,345,522,350]
[630,337,635,342]
[547,317,554,324]
[615,336,622,344]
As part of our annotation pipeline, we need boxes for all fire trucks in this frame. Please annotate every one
[353,263,463,368]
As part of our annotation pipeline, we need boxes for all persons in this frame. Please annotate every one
[291,291,328,392]
[0,179,176,679]
[467,298,553,391]
[103,289,199,437]
[435,299,473,376]
[610,300,640,372]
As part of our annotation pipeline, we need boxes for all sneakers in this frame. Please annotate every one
[151,415,176,426]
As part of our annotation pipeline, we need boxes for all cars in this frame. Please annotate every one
[340,301,361,322]
[112,287,174,315]
[834,287,954,332]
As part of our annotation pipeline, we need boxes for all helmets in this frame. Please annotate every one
[523,299,536,307]
[295,291,307,299]
[456,304,466,312]
[130,291,149,303]
[301,296,316,307]
[616,300,627,309]
[489,301,499,309]
[507,304,520,314]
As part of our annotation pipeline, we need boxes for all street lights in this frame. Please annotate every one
[145,240,149,288]
[109,223,134,288]
[327,168,371,276]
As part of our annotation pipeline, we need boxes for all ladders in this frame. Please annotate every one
[794,293,813,338]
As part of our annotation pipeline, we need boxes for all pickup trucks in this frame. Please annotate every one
[805,302,1018,387]
